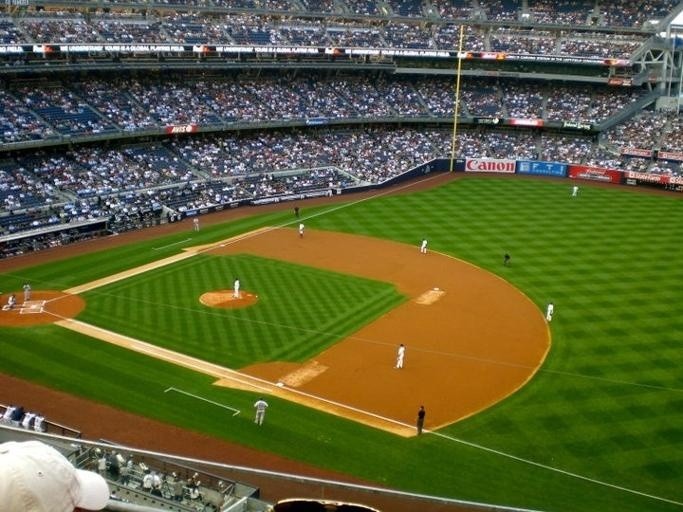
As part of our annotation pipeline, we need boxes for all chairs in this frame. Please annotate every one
[1,0,683,258]
[60,442,232,512]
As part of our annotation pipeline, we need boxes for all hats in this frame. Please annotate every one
[0,440,109,512]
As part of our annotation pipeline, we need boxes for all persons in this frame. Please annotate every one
[3,404,46,432]
[234,278,239,296]
[0,440,202,512]
[8,294,16,305]
[396,344,406,369]
[416,405,425,434]
[504,253,510,267]
[0,1,682,259]
[23,282,31,300]
[546,302,554,322]
[254,398,268,426]
